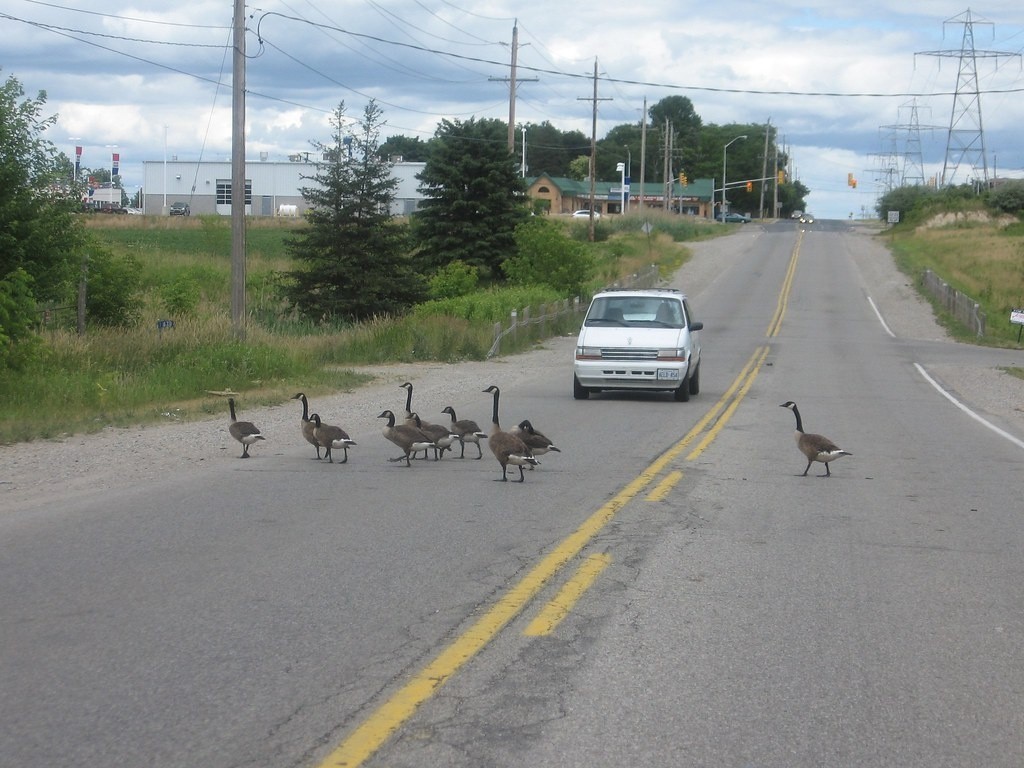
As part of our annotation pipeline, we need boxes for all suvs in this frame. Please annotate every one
[169,202,191,216]
[573,288,704,401]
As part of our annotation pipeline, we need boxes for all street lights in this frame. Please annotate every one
[519,127,527,186]
[722,135,748,224]
[104,144,119,210]
[624,144,631,210]
[69,138,81,199]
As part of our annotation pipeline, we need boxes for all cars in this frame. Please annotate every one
[799,214,815,223]
[717,212,751,223]
[791,210,803,220]
[571,210,601,221]
[79,203,143,215]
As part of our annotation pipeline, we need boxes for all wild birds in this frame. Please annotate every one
[778,400,853,477]
[227,380,563,484]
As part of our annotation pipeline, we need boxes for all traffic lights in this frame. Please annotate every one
[747,182,752,192]
[679,173,684,184]
[853,180,857,188]
[682,177,688,186]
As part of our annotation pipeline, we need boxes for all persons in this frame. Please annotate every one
[655,303,674,323]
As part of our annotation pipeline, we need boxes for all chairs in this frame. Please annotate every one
[600,307,624,321]
[657,309,675,322]
